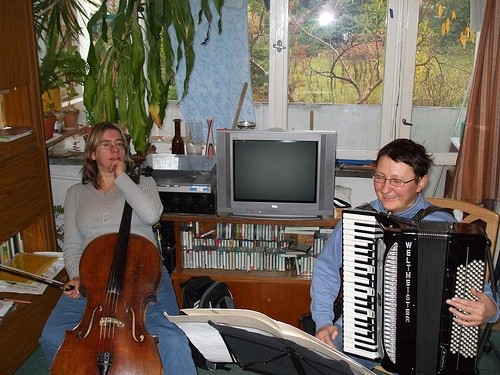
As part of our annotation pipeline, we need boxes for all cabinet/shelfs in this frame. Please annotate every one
[159,213,341,327]
[0,0,64,375]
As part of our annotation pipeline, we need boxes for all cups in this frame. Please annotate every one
[185,120,203,154]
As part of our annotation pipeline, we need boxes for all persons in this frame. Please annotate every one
[310,138,500,375]
[39,121,197,375]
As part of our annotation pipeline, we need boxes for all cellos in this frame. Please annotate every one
[50,150,165,375]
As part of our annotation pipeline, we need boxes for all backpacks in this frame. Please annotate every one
[180,274,236,310]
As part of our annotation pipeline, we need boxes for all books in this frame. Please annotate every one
[0,231,24,265]
[0,252,57,286]
[0,126,33,142]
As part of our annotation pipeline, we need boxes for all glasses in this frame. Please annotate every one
[370,170,417,187]
[96,141,127,150]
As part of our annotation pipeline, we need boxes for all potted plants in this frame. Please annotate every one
[35,0,224,154]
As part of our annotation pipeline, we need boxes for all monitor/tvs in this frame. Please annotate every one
[215,129,337,221]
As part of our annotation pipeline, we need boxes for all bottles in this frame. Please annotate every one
[171,119,184,154]
[205,119,215,157]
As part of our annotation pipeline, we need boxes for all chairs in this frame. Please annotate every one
[375,198,500,375]
